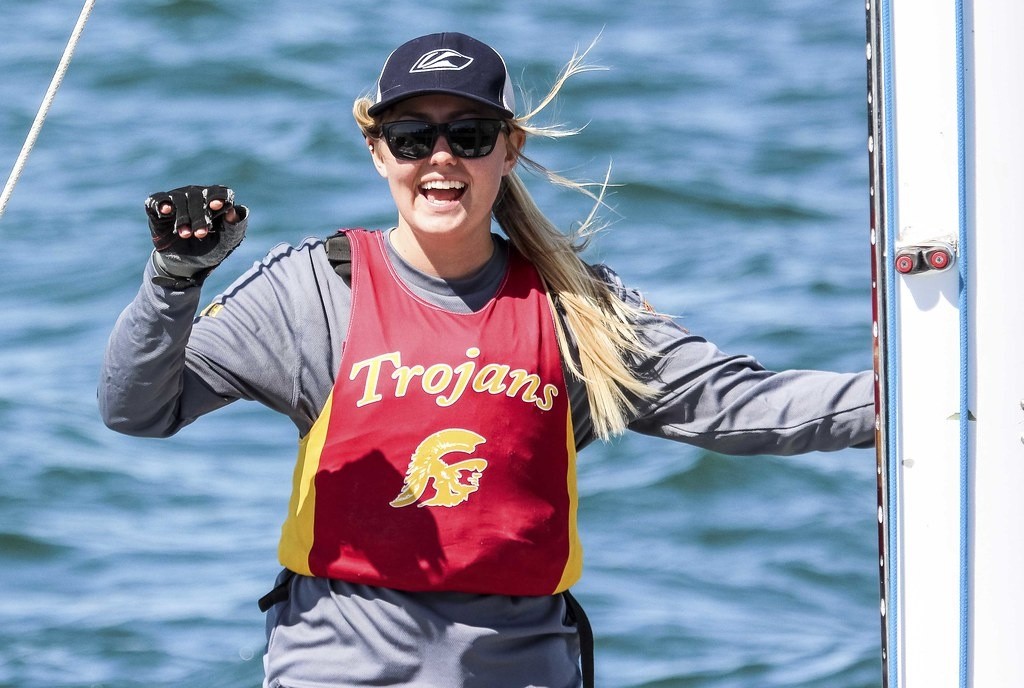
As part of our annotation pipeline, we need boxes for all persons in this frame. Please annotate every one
[98,31,877,688]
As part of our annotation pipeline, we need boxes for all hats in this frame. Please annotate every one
[367,31,515,120]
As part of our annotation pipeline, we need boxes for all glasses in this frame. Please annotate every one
[366,115,511,160]
